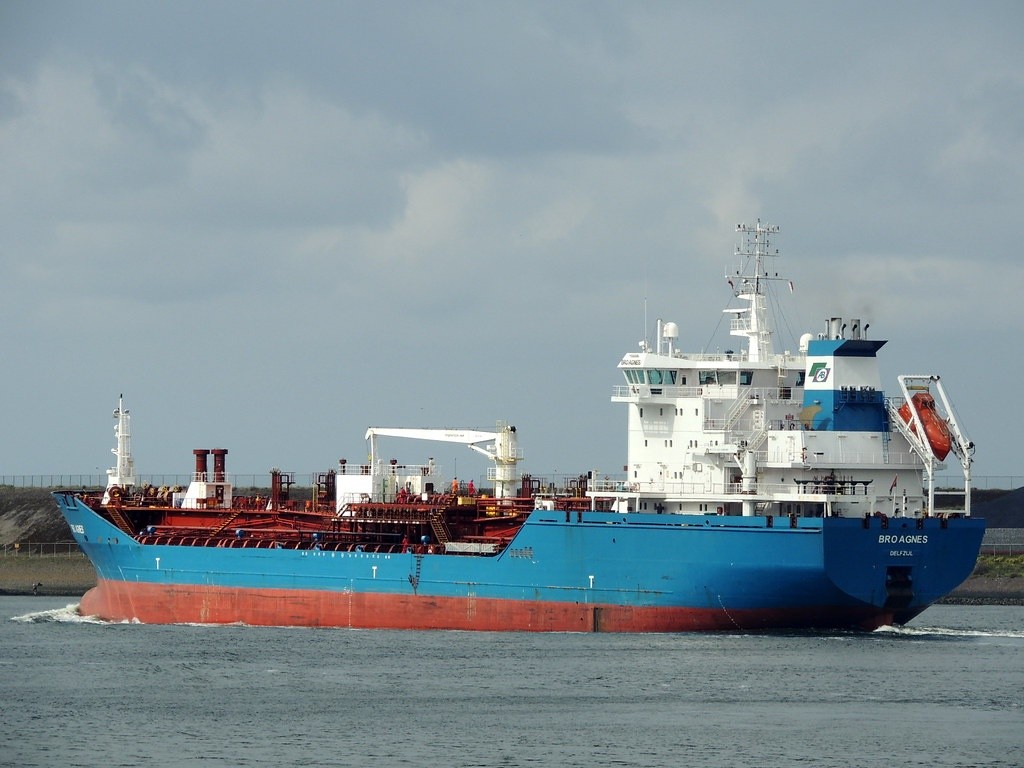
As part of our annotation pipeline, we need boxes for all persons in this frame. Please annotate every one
[469,480,475,496]
[400,487,405,504]
[403,535,408,553]
[453,478,458,492]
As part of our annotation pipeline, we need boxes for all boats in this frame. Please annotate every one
[48,213,988,634]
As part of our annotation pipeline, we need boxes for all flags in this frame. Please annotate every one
[890,476,897,495]
